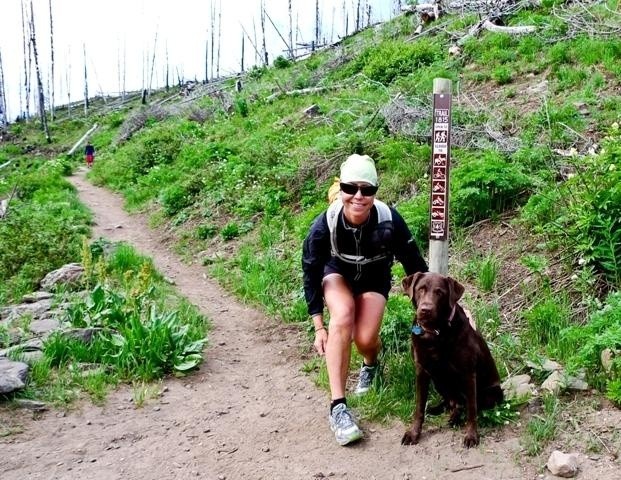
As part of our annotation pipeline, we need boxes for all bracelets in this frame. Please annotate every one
[315,326,328,334]
[311,313,323,318]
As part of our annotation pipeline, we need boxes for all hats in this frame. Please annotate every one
[338,184,378,195]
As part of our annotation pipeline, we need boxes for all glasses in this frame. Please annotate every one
[340,155,378,185]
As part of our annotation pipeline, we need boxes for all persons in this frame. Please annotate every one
[236,77,242,91]
[85,142,95,168]
[302,153,476,446]
[422,13,435,22]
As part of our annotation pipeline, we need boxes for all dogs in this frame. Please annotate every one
[400,270,504,450]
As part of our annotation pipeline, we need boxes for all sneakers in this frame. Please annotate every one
[356,359,380,396]
[330,403,363,446]
